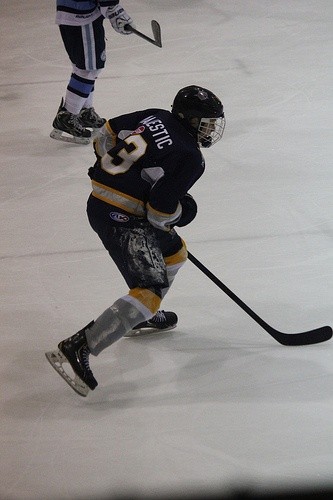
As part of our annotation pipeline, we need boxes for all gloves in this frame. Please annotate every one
[105,6,135,36]
[175,193,197,228]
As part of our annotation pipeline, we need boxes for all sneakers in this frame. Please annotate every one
[75,107,106,131]
[45,319,98,397]
[124,310,177,338]
[50,97,91,146]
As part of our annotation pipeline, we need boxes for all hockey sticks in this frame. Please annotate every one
[185,250,333,347]
[125,20,162,48]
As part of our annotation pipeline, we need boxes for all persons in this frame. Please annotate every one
[49,0,137,145]
[46,85,227,398]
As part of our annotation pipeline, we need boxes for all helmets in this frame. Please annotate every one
[171,85,226,147]
